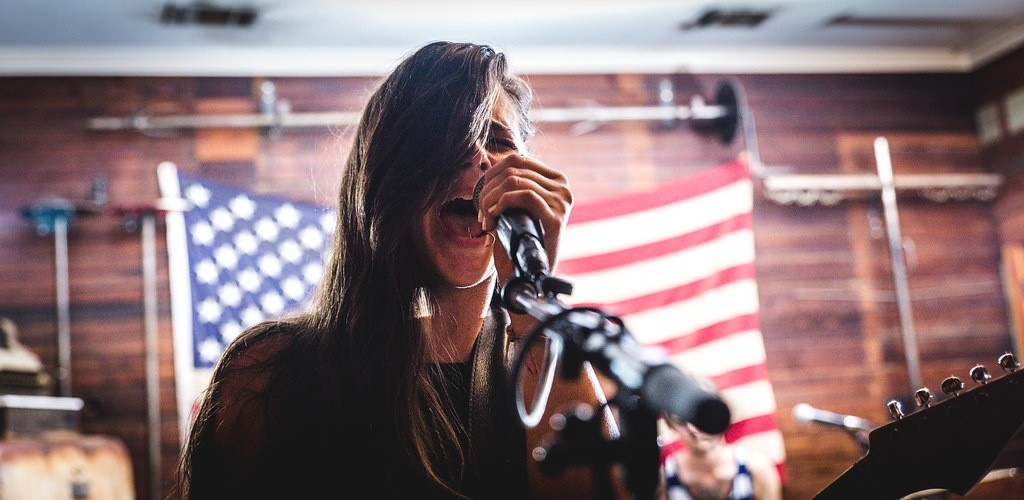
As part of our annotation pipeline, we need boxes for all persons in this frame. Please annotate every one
[183,41,668,500]
[666,375,782,500]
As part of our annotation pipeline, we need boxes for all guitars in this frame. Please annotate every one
[811,352,1023,500]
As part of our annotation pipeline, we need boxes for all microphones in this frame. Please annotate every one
[473,171,549,284]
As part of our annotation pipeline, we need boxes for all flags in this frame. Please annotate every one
[156,162,788,485]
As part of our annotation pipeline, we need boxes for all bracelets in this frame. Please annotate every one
[503,325,546,375]
